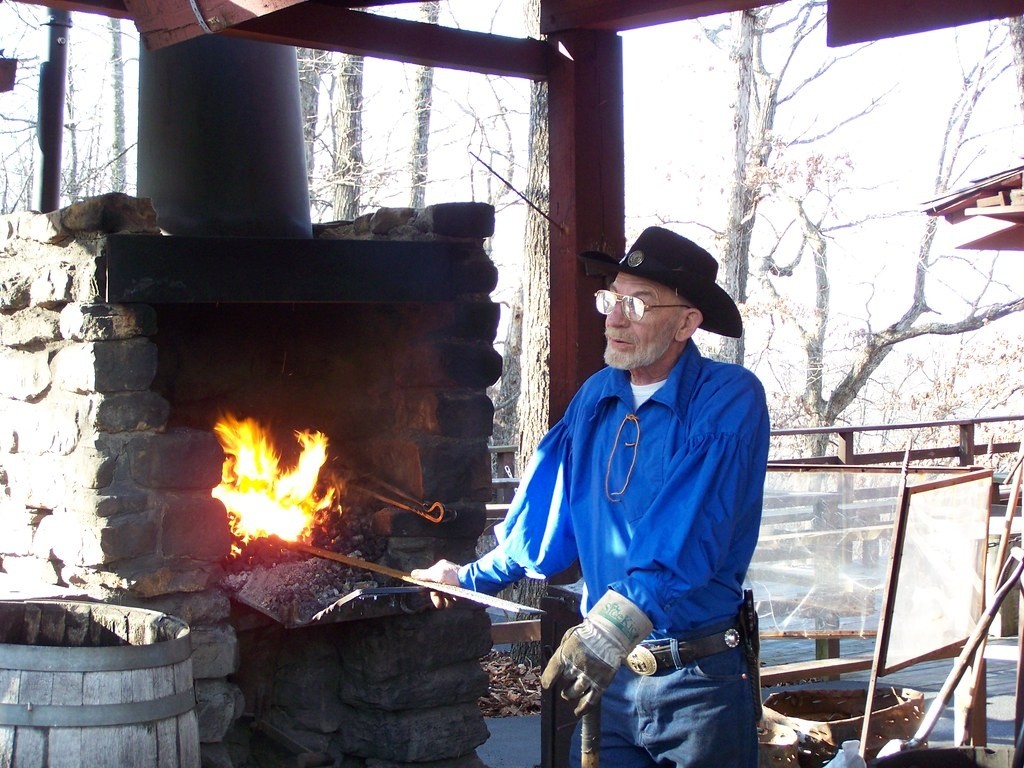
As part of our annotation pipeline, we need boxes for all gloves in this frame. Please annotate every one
[540,589,653,718]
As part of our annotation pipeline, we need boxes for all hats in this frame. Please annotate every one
[579,226,743,338]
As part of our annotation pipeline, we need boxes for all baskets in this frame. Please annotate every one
[762,688,928,768]
[757,721,801,768]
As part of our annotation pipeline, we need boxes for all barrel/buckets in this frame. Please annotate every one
[0,598,203,768]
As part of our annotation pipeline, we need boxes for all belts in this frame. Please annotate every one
[621,626,745,676]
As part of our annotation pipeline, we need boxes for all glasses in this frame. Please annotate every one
[594,289,690,322]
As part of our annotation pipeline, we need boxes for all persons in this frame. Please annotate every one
[410,226,771,768]
